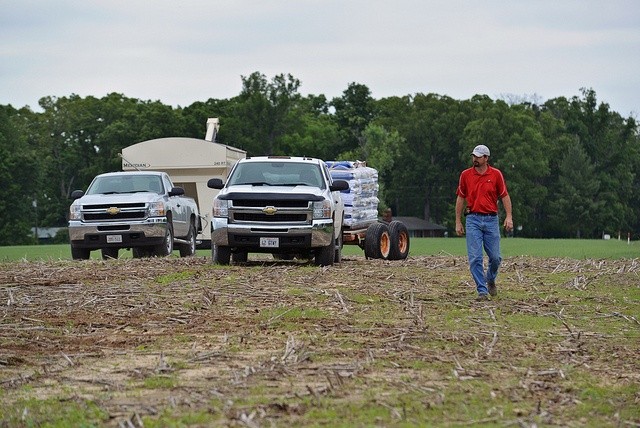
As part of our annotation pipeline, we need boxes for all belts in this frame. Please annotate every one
[469,212,496,216]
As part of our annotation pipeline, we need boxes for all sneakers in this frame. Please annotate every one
[476,294,488,301]
[488,283,497,296]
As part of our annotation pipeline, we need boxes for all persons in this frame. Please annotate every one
[454,145,513,300]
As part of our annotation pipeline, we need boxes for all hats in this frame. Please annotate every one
[469,144,491,158]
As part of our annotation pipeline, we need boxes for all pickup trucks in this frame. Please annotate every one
[68,171,203,261]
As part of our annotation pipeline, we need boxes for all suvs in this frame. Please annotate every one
[206,154,351,266]
